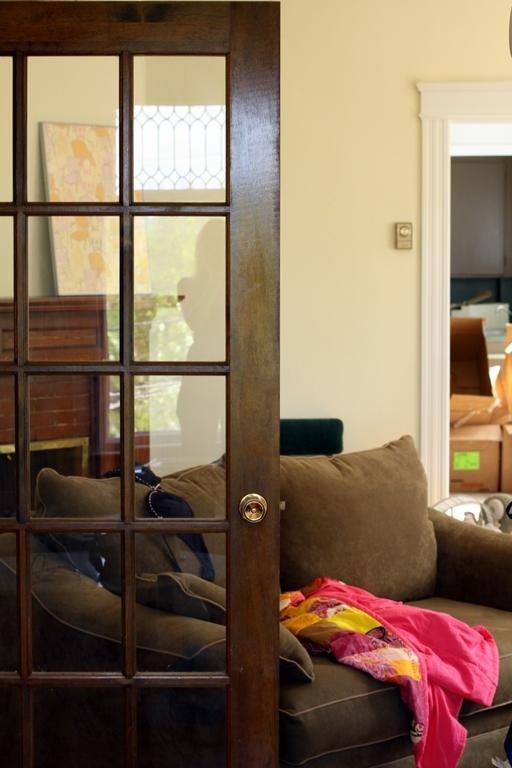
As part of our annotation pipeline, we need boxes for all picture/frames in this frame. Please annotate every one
[39,121,147,296]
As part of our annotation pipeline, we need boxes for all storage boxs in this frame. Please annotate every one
[496,322,511,423]
[502,425,512,496]
[451,315,496,424]
[450,423,502,495]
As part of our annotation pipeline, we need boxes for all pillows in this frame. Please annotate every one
[279,435,436,600]
[138,572,316,683]
[150,449,226,588]
[36,466,164,583]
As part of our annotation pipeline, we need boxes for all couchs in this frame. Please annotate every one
[0,508,510,767]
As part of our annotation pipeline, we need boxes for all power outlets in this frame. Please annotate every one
[396,222,413,250]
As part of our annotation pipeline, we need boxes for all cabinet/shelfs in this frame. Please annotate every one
[105,431,187,474]
[0,297,105,476]
